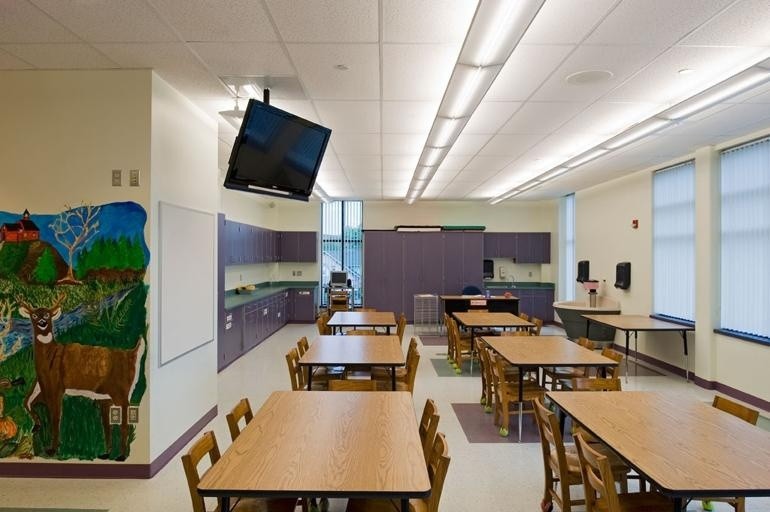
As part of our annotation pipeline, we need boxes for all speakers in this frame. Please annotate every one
[348,279,352,288]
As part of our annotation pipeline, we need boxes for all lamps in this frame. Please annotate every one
[219,77,264,133]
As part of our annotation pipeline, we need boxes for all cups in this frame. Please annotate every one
[485,290,490,298]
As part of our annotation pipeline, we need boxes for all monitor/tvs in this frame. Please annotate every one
[330,271,348,287]
[224,98,332,202]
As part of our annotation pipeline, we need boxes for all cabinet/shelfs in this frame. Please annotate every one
[439,295,520,317]
[485,282,555,324]
[217,213,317,372]
[484,232,516,258]
[515,232,551,264]
[362,230,485,322]
